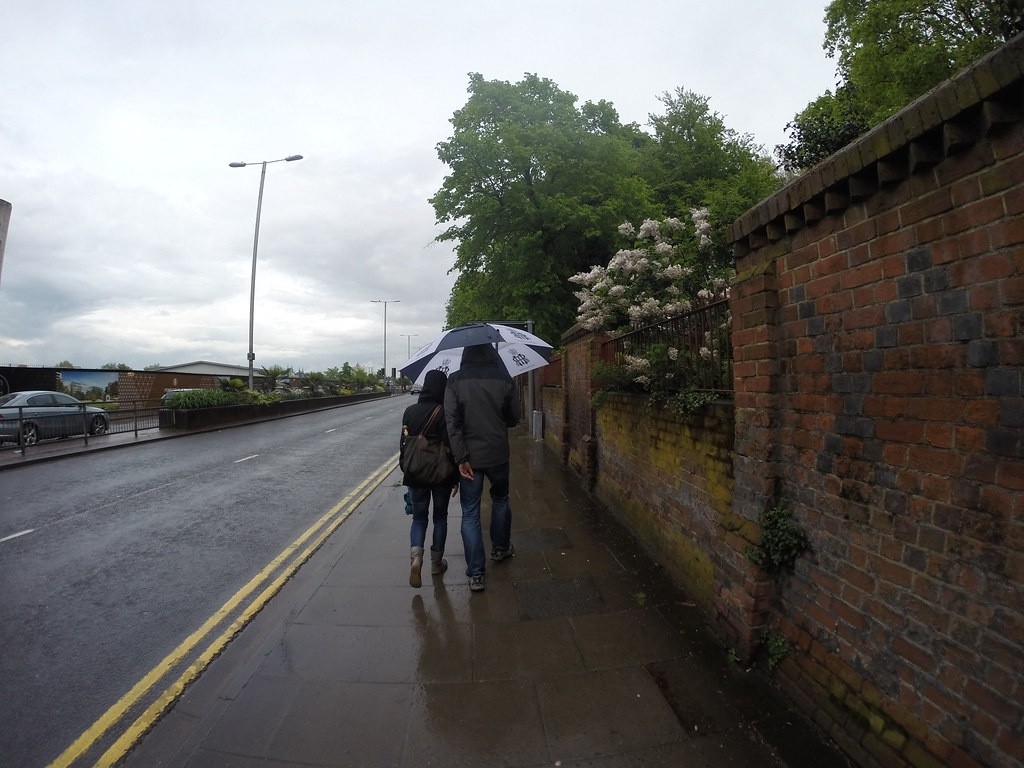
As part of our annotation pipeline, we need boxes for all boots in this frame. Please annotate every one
[409,546,425,588]
[429,545,448,574]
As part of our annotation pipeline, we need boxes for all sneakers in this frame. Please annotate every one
[469,570,485,590]
[489,541,514,560]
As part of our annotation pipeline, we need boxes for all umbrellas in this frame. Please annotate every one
[396,322,553,386]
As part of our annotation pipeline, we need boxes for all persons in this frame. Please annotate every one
[400,369,460,588]
[443,342,521,589]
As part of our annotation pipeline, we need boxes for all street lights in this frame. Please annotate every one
[400,334,418,360]
[370,300,400,386]
[227,154,305,394]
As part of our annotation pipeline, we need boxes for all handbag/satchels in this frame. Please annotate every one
[402,404,459,489]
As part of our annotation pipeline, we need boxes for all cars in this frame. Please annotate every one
[0,390,110,448]
[157,388,207,409]
